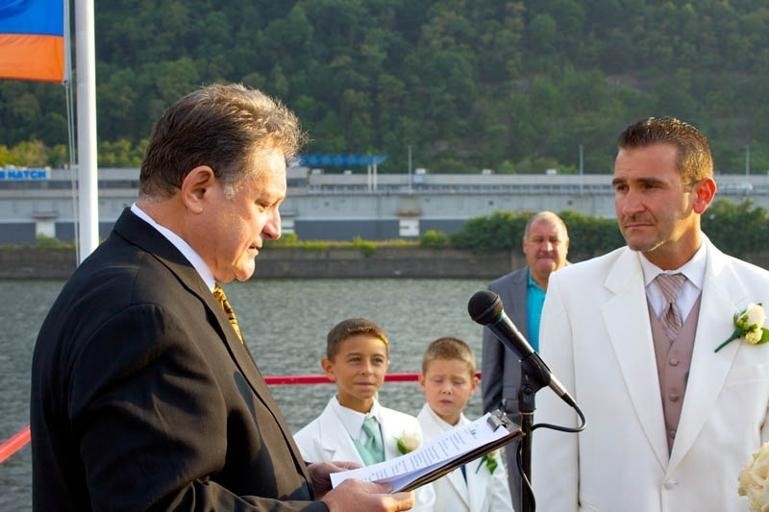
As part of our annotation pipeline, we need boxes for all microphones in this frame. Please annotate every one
[468,291,576,409]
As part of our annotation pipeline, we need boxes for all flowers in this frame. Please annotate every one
[395,432,420,452]
[475,450,496,476]
[714,303,769,353]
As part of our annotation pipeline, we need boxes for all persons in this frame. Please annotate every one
[291,317,435,512]
[32,83,415,512]
[530,117,769,512]
[481,211,573,512]
[415,337,514,512]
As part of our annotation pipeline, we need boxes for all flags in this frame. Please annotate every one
[0,0,65,83]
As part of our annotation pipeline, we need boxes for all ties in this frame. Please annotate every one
[656,274,686,342]
[213,282,243,345]
[363,418,384,462]
